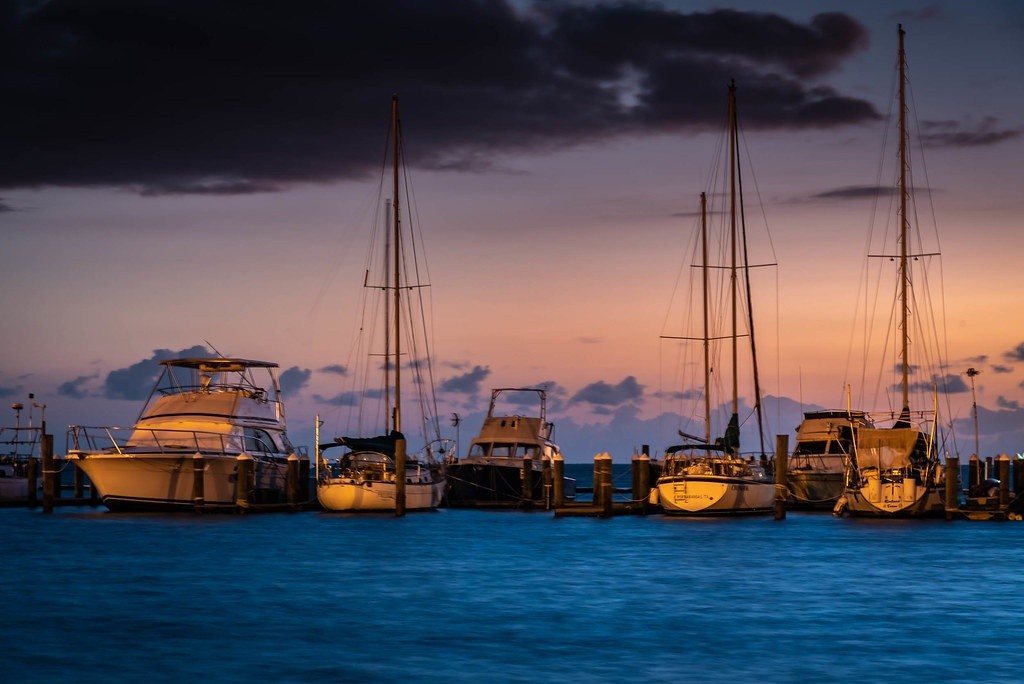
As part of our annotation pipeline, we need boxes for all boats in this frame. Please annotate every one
[776,364,879,512]
[0,391,63,506]
[68,339,313,510]
[444,387,579,509]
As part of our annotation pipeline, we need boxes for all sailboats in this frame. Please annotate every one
[647,108,792,513]
[313,118,454,510]
[830,21,963,519]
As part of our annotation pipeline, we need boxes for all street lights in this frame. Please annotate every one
[965,368,984,485]
